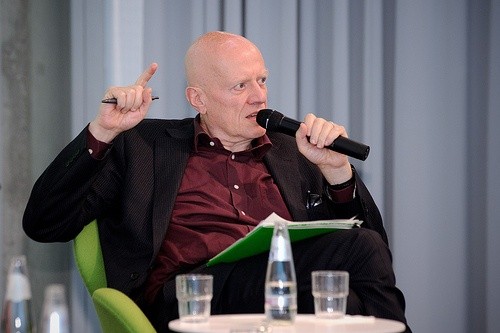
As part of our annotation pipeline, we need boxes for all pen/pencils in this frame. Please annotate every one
[102,95,160,103]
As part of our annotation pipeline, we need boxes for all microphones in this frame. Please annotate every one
[255,108,369,160]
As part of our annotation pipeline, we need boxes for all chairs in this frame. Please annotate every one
[75,221,158,333]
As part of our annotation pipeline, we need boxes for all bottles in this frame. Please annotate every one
[37,285,69,333]
[264,221,298,324]
[4,255,33,333]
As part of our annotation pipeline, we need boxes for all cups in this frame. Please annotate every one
[311,270,349,318]
[175,274,213,323]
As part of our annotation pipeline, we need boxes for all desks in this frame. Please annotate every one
[168,313,404,333]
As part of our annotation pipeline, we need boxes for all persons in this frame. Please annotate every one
[22,31,413,333]
